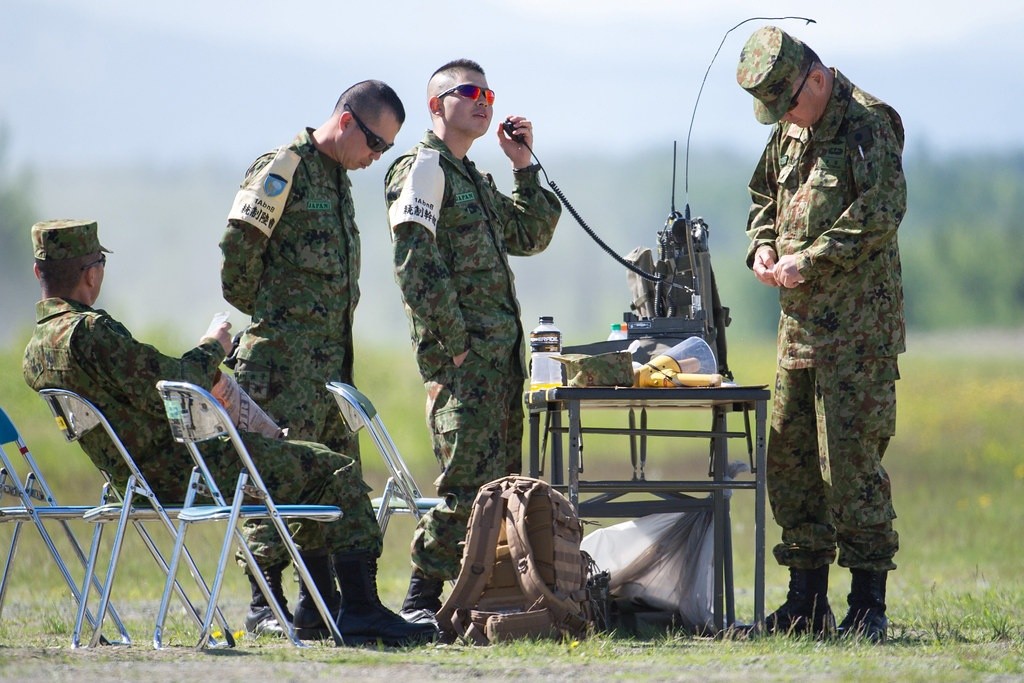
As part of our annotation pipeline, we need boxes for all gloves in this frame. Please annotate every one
[222,326,249,370]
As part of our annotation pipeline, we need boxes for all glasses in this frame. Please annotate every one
[81,254,106,270]
[785,60,813,113]
[437,85,495,105]
[344,104,395,154]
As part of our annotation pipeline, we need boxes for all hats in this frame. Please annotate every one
[737,26,804,126]
[32,219,114,261]
[549,352,634,388]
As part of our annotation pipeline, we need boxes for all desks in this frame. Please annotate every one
[523,385,772,641]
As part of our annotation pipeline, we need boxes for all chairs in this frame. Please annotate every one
[153,379,344,652]
[37,389,237,650]
[0,406,133,646]
[325,380,446,538]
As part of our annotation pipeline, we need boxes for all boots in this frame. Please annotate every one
[830,567,887,644]
[333,551,436,647]
[734,563,836,642]
[248,559,293,639]
[292,551,340,640]
[397,561,458,644]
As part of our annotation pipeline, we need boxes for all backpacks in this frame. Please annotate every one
[436,474,595,646]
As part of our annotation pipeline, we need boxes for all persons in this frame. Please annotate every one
[383,61,561,620]
[25,219,437,645]
[219,79,407,639]
[737,26,907,643]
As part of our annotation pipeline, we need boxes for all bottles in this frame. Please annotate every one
[529,316,562,390]
[607,324,627,341]
